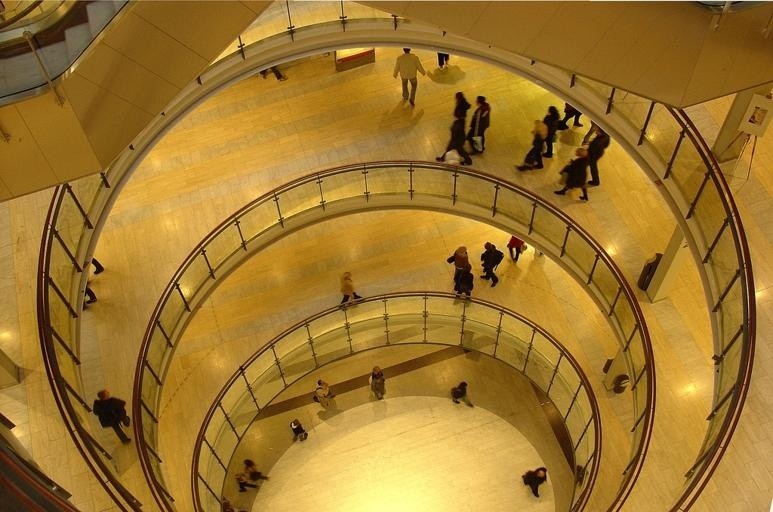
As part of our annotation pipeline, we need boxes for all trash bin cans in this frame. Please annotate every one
[613,375,630,394]
[603,359,613,374]
[638,253,663,291]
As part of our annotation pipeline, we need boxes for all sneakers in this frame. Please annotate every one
[543,151,553,159]
[435,146,487,166]
[553,180,600,201]
[573,122,584,127]
[480,274,499,287]
[516,164,543,171]
[403,96,415,106]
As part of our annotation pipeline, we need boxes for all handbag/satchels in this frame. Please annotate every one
[556,119,569,131]
[471,135,484,153]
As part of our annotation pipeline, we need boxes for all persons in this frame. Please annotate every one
[436,51,450,72]
[367,365,386,400]
[243,459,268,481]
[450,381,473,407]
[91,389,131,443]
[453,263,474,307]
[315,380,335,405]
[339,271,362,311]
[222,495,234,511]
[479,241,498,288]
[523,466,546,498]
[392,47,426,106]
[289,419,308,442]
[234,472,257,492]
[434,81,612,203]
[259,66,287,82]
[451,246,468,290]
[505,236,525,264]
